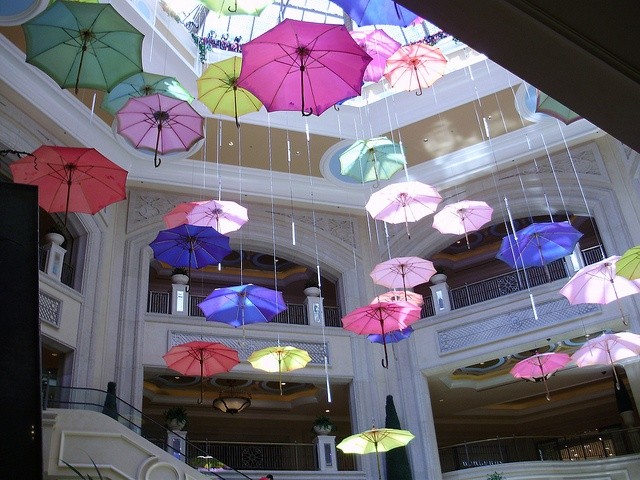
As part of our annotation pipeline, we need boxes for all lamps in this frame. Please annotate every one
[213,385,251,416]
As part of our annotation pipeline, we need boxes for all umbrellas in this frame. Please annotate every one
[246,343,313,397]
[615,244,640,281]
[163,201,213,230]
[195,282,288,346]
[236,17,373,117]
[431,200,494,250]
[195,55,264,128]
[332,81,365,105]
[198,0,276,17]
[185,200,249,234]
[338,135,407,188]
[571,330,640,390]
[364,180,443,240]
[335,423,415,480]
[162,335,241,404]
[21,0,146,95]
[367,325,416,361]
[383,42,447,96]
[98,72,194,117]
[347,29,400,83]
[510,351,572,400]
[340,301,422,368]
[147,224,232,292]
[9,144,128,232]
[494,221,586,267]
[329,0,418,28]
[533,89,585,127]
[371,291,426,306]
[113,92,204,168]
[368,256,438,301]
[557,254,640,327]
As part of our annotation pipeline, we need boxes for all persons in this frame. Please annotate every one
[258,474,274,480]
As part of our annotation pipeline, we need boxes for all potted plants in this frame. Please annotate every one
[612,368,635,427]
[309,414,338,435]
[162,405,189,432]
